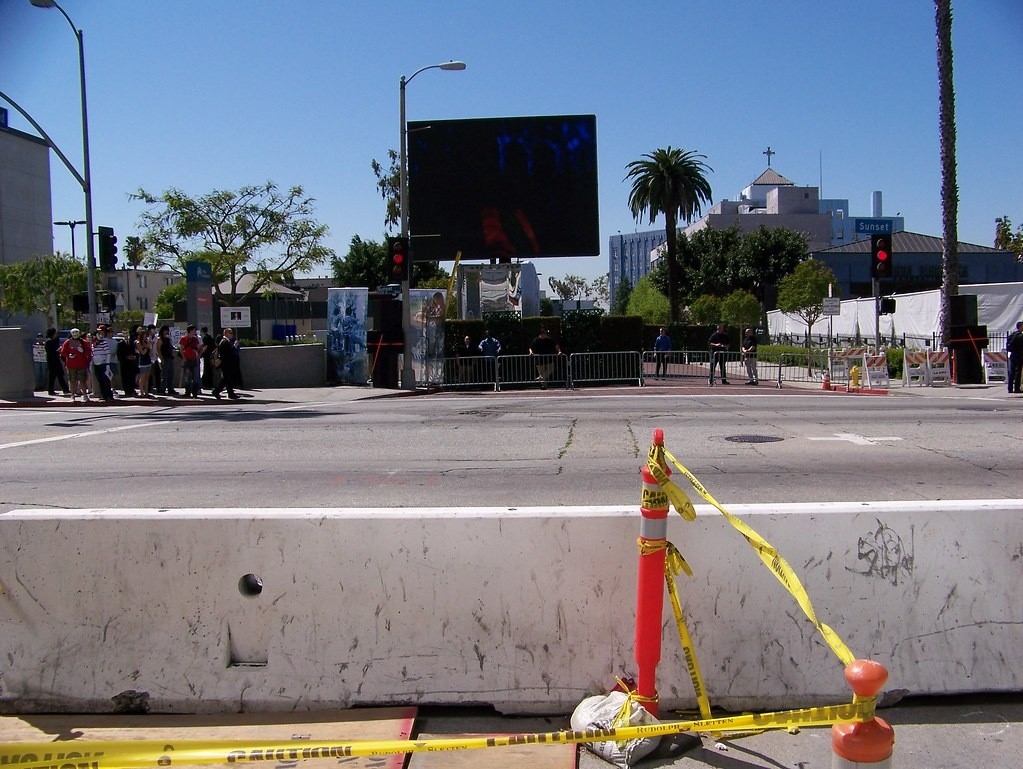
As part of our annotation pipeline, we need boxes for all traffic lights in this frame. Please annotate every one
[99,225,118,273]
[102,293,116,311]
[881,298,897,314]
[388,237,408,282]
[871,233,893,278]
[73,293,88,312]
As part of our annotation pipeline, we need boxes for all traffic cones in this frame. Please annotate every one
[820,367,833,389]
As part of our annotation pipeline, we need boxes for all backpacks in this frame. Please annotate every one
[1006,331,1022,352]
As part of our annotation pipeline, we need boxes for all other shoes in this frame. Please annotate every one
[751,381,758,385]
[1008,389,1013,393]
[212,390,220,399]
[1013,389,1023,393]
[662,376,666,380]
[655,376,661,380]
[745,381,754,385]
[228,394,241,398]
[722,381,730,385]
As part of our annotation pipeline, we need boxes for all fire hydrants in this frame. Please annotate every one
[849,365,860,387]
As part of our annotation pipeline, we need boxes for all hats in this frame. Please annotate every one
[70,328,80,336]
[96,325,107,331]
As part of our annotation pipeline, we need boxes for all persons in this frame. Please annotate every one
[529,329,562,390]
[654,328,671,380]
[708,325,730,384]
[1007,322,1023,393]
[331,307,357,356]
[33,323,241,402]
[456,329,502,387]
[742,329,758,384]
[425,292,446,360]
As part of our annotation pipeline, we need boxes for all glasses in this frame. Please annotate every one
[107,330,114,334]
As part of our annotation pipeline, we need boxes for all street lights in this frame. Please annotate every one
[399,60,467,391]
[29,0,101,396]
[53,220,86,260]
